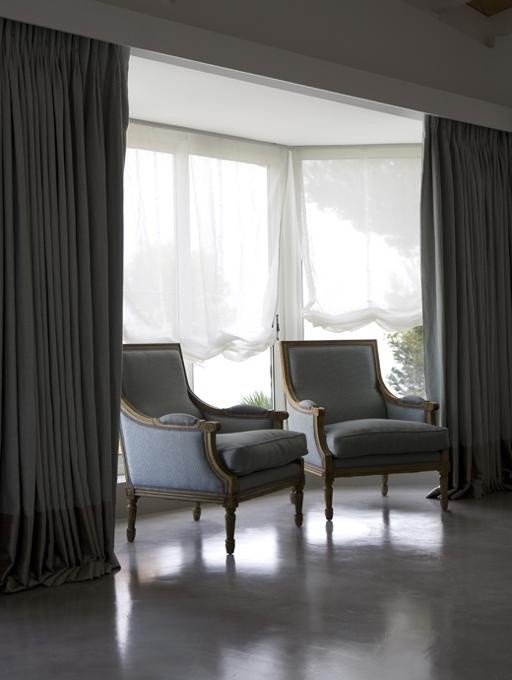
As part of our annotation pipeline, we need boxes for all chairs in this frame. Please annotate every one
[118,342,309,555]
[278,339,451,520]
